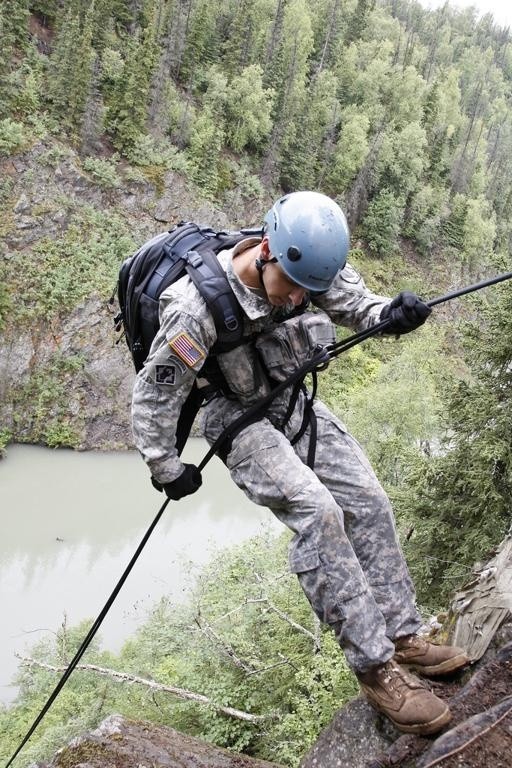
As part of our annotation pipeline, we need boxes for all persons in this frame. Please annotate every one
[126,186,472,735]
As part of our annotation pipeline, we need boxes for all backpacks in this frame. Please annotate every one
[109,219,263,373]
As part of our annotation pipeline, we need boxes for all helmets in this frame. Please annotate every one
[264,190,352,293]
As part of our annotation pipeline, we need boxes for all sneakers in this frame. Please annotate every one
[393,636,471,676]
[355,656,452,735]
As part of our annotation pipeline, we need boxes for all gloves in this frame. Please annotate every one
[164,463,202,501]
[381,292,431,340]
